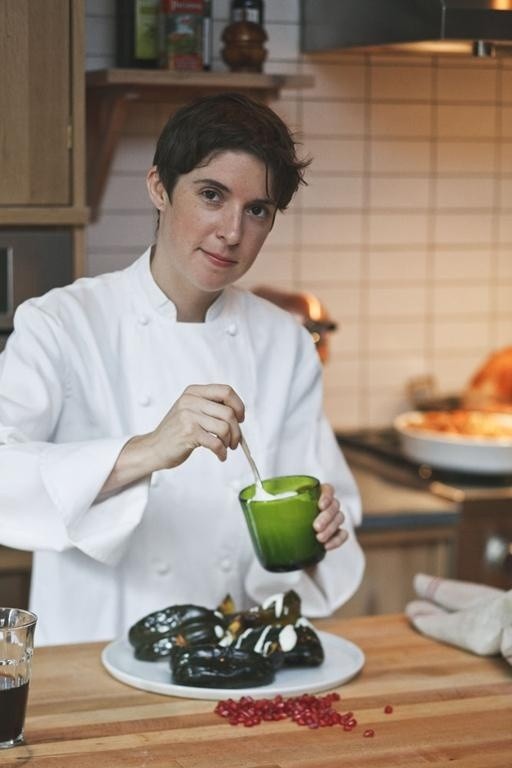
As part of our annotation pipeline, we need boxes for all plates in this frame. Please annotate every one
[102,633,363,696]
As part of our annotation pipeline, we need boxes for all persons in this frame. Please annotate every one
[0,91,366,648]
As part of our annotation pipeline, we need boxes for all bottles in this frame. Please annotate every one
[119,0,160,68]
[229,0,265,28]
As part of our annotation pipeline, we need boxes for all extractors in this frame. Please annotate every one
[297,3,512,69]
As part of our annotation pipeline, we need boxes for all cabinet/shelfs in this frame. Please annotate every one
[0,0,92,226]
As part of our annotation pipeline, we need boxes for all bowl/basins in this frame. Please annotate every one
[396,409,512,478]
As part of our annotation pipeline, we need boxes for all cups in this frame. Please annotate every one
[1,608,40,748]
[239,475,324,570]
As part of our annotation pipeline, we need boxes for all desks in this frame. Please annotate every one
[0,611,512,767]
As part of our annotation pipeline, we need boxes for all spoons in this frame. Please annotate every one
[225,435,275,502]
